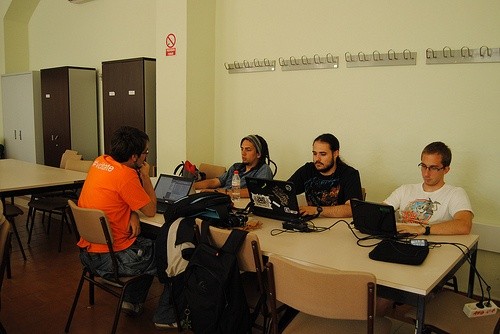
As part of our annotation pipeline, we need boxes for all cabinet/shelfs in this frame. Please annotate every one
[40,66,98,168]
[101,57,156,177]
[0,71,43,199]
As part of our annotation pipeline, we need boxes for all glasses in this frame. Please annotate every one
[418,163,444,173]
[141,147,152,155]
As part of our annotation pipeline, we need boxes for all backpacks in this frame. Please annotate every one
[175,220,269,334]
[164,190,241,228]
[173,160,207,182]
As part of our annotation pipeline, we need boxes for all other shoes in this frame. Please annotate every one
[154,322,177,330]
[121,301,145,316]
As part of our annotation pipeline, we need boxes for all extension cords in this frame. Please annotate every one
[464,299,498,318]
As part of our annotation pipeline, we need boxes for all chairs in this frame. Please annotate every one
[0,150,459,334]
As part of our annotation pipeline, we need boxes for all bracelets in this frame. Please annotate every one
[225,189,227,195]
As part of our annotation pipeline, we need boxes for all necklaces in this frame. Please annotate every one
[214,188,220,192]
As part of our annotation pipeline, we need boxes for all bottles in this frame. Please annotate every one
[231,170,241,203]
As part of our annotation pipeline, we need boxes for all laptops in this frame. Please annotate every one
[245,176,319,224]
[349,198,419,239]
[153,174,194,214]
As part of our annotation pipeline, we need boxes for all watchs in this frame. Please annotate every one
[315,206,322,217]
[422,223,432,235]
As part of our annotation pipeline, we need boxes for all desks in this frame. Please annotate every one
[0,159,88,216]
[135,176,481,334]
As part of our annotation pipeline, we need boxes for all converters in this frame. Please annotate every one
[410,238,428,247]
[282,221,309,231]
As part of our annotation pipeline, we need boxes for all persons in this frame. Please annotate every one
[76,126,183,328]
[194,135,273,197]
[379,142,474,235]
[286,133,363,217]
